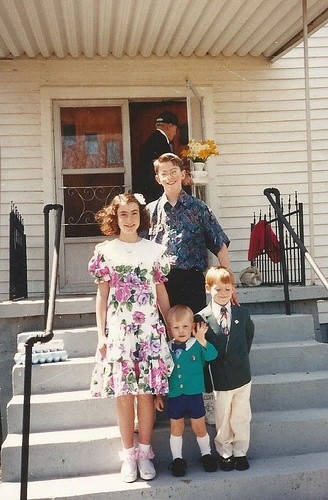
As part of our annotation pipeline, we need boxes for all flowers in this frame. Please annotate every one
[180,137,217,162]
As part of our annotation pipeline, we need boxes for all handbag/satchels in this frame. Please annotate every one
[239,266,263,286]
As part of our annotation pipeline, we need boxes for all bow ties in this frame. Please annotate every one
[172,343,186,351]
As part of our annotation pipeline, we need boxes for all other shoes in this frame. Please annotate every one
[203,392,217,426]
[199,451,217,472]
[168,458,187,477]
[120,454,137,482]
[217,452,235,471]
[137,452,156,480]
[235,456,249,471]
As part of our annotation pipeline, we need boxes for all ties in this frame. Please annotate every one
[221,306,229,336]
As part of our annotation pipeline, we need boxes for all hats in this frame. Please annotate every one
[155,111,182,130]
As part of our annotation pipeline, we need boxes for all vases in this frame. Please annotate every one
[192,163,206,172]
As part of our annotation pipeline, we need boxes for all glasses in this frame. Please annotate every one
[158,169,181,177]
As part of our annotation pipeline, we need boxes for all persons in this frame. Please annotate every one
[193,266,254,472]
[132,111,194,209]
[134,153,241,433]
[154,304,218,477]
[88,193,172,483]
